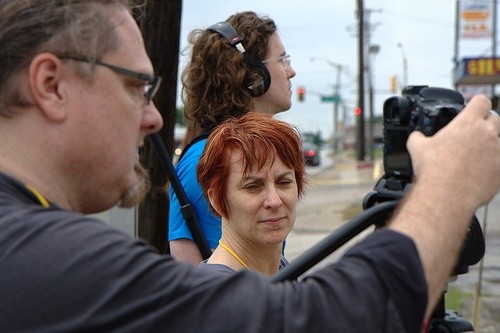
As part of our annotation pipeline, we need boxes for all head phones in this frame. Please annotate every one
[206,22,271,98]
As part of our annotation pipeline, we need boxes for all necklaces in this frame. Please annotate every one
[219,239,249,269]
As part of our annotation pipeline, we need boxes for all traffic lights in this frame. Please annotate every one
[354,107,360,115]
[298,85,303,101]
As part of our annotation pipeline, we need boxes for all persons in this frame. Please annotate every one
[168,10,296,271]
[199,105,313,283]
[0,0,500,333]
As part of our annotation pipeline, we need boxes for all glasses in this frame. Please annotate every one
[26,52,162,105]
[262,54,291,72]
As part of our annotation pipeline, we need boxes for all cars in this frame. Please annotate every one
[302,142,321,167]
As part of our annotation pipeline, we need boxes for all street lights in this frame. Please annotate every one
[396,42,407,87]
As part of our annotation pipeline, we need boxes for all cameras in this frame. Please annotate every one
[384,84,466,178]
